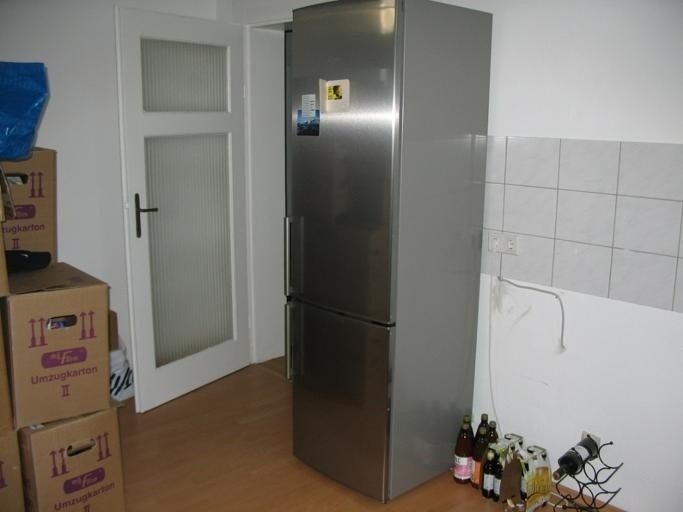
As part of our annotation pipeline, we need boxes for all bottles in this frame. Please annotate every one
[453,414,601,502]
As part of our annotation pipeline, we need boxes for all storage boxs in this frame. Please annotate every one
[108,311,138,438]
[0,146,60,265]
[17,395,124,511]
[1,261,110,429]
[0,428,25,512]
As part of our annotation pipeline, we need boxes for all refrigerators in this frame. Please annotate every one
[283,1,493,503]
